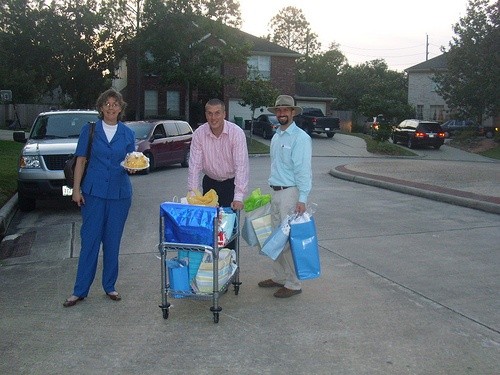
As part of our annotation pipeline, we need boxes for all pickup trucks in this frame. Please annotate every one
[293,108,340,138]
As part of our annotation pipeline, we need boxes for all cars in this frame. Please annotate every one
[390,119,444,150]
[372,114,394,133]
[13,110,100,211]
[253,114,280,139]
[440,119,485,139]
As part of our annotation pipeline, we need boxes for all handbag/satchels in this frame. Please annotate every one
[166,248,232,299]
[241,188,320,280]
[64,121,96,189]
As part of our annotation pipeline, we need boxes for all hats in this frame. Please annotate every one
[267,94,302,114]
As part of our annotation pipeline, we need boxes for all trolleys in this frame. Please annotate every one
[158,202,241,323]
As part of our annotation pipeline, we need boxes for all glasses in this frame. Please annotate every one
[105,103,117,107]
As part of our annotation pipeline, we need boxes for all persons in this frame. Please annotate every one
[187,100,249,293]
[63,89,138,307]
[258,96,311,298]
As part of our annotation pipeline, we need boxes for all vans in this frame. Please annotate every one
[122,119,193,175]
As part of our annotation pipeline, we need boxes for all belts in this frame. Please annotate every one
[270,185,288,191]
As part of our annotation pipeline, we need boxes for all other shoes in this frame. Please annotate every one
[257,278,284,287]
[105,291,121,301]
[63,294,84,306]
[273,287,302,298]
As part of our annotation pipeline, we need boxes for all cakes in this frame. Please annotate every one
[124,151,150,168]
